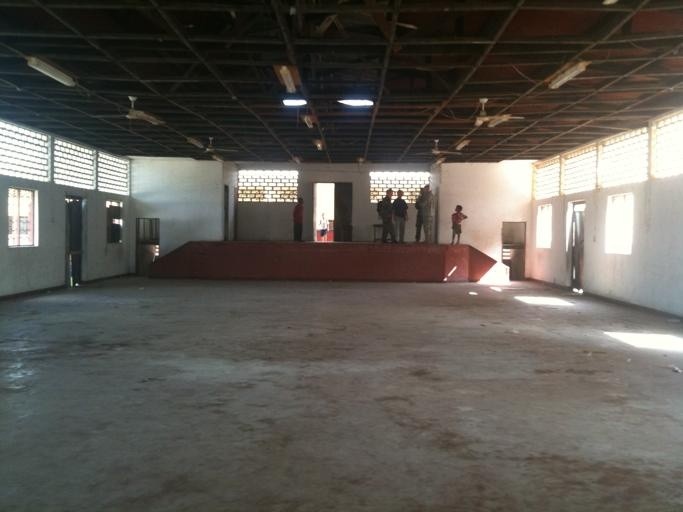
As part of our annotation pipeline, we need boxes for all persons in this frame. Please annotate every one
[415,188,426,243]
[450,204,468,246]
[376,189,398,244]
[419,184,434,244]
[293,196,304,241]
[392,190,409,244]
[318,211,330,241]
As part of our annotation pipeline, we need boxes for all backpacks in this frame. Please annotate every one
[377,201,385,216]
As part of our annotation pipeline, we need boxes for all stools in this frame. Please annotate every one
[373,224,383,242]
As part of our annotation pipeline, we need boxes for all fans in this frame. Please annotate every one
[187,136,239,154]
[90,95,167,125]
[416,139,462,156]
[449,98,526,127]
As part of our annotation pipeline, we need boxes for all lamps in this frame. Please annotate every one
[303,113,313,129]
[27,55,76,87]
[548,60,591,90]
[279,64,296,94]
